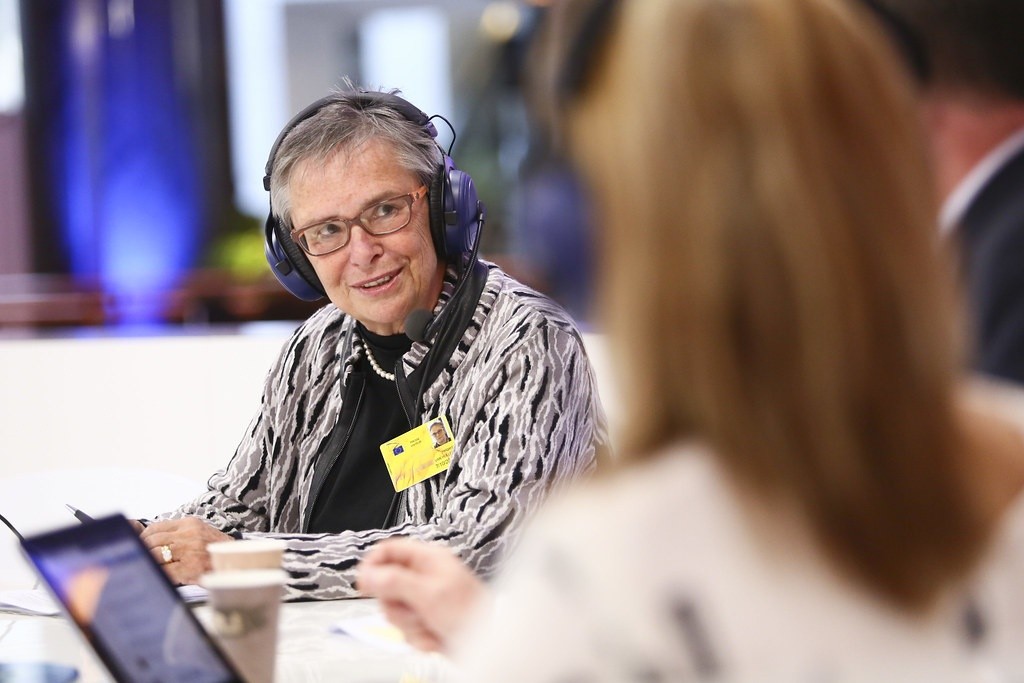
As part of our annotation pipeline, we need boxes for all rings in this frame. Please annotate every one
[159,545,173,563]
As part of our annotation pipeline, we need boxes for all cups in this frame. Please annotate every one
[205,540,289,576]
[198,568,287,683]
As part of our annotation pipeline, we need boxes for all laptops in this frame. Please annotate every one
[17,512,253,683]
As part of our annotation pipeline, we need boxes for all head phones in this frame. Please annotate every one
[265,91,479,301]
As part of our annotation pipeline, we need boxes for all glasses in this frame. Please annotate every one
[290,184,428,258]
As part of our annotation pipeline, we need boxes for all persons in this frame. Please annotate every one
[348,0,1021,681]
[124,85,609,586]
[856,0,1023,387]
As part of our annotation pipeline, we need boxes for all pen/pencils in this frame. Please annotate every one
[65,503,93,523]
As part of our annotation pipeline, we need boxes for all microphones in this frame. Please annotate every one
[403,213,485,344]
